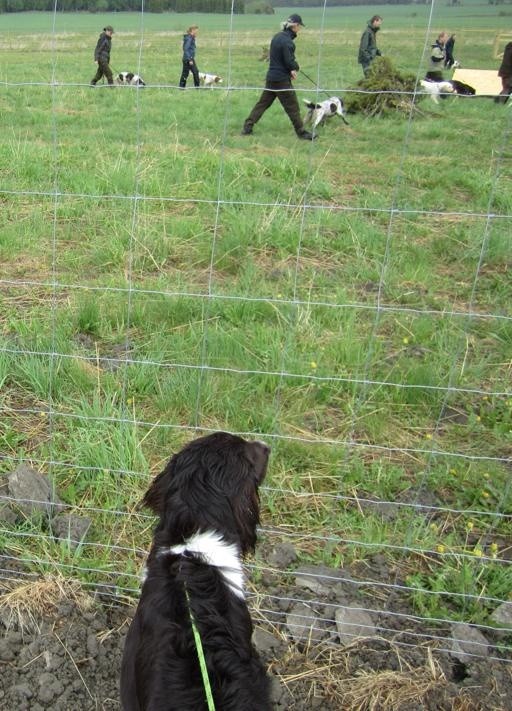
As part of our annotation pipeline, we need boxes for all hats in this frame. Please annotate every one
[287,13,306,27]
[103,26,116,34]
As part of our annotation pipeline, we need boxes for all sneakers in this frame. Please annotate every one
[298,130,318,141]
[241,127,254,136]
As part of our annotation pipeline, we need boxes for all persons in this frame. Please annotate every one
[445,34,456,70]
[89,25,116,90]
[493,42,512,105]
[241,13,318,140]
[358,16,383,79]
[177,25,202,90]
[426,31,448,99]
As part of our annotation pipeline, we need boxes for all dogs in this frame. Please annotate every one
[440,81,475,99]
[302,96,350,138]
[190,72,223,92]
[420,79,454,104]
[120,432,275,711]
[447,60,460,70]
[113,72,145,88]
